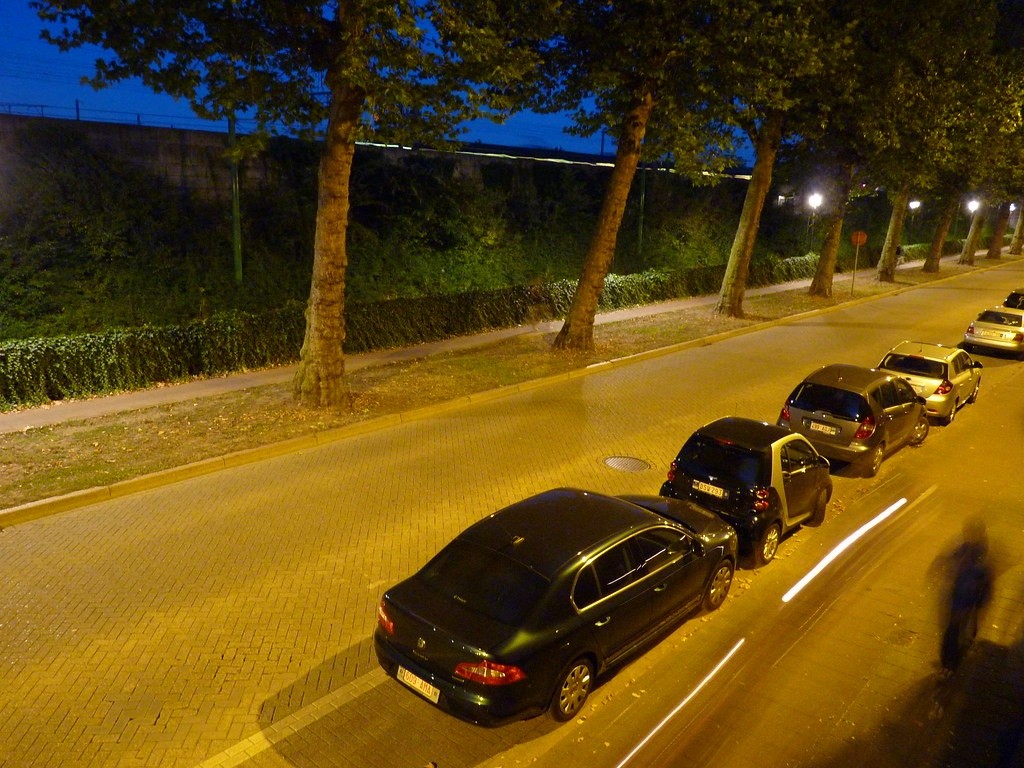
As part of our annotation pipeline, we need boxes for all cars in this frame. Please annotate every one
[374,485,739,729]
[658,416,835,569]
[777,363,930,478]
[1003,288,1024,310]
[874,340,984,425]
[962,306,1024,352]
